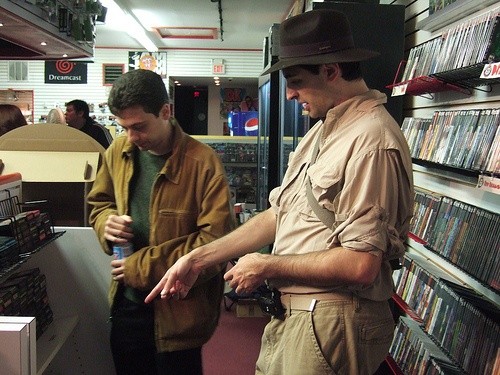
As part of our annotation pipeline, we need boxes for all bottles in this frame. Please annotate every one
[113,235,134,260]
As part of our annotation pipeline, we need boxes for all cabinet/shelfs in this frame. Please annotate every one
[379,0,499,374]
[1,171,115,375]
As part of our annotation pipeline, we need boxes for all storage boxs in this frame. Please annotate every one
[224,293,267,317]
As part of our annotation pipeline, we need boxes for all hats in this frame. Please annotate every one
[260,10,381,76]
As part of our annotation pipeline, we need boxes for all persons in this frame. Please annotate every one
[145,10,414,375]
[63,99,113,151]
[46,107,67,125]
[84,68,231,375]
[0,104,28,140]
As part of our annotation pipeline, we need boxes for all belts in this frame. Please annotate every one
[278,290,366,313]
